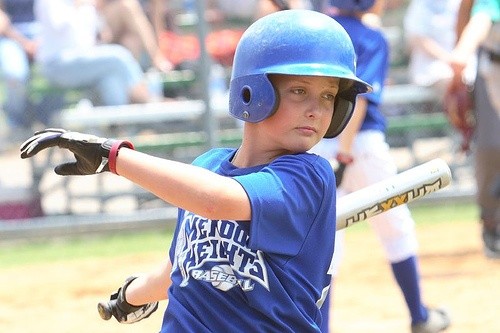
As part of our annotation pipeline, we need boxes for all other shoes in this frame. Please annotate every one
[412,309,449,333]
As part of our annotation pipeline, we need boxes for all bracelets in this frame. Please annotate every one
[336,153,353,163]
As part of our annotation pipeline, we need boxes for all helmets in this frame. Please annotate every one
[329,0,376,11]
[228,9,373,139]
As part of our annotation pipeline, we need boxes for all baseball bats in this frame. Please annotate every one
[95,155,456,322]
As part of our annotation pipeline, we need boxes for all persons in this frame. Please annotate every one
[258,0,500,333]
[0,0,178,134]
[21,9,372,333]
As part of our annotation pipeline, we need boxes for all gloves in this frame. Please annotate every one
[20,128,134,176]
[114,277,159,324]
